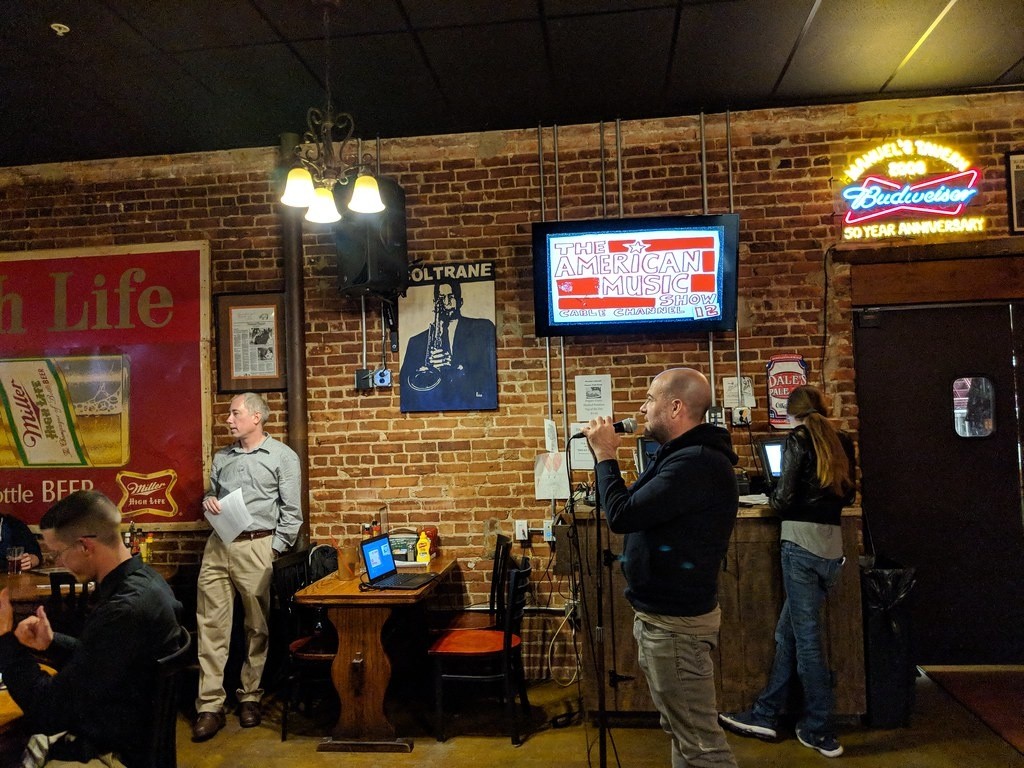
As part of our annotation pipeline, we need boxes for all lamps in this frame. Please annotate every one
[281,0,386,224]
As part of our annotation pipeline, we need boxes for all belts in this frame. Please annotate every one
[237,531,272,539]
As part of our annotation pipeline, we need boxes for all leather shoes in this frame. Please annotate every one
[194,709,226,738]
[240,702,261,727]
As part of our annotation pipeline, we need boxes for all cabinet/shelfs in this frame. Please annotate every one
[561,505,868,718]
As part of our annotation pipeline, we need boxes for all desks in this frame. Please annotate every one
[1,566,174,603]
[295,556,456,753]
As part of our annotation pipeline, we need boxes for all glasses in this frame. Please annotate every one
[48,535,96,560]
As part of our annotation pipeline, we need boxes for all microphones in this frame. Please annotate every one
[572,418,637,438]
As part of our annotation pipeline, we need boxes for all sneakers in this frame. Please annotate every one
[720,711,777,739]
[796,721,843,757]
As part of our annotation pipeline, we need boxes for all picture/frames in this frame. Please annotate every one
[1005,150,1024,236]
[213,290,287,395]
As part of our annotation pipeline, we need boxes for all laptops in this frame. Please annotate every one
[360,533,439,589]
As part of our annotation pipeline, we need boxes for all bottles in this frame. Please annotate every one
[416,532,431,563]
[124,528,153,563]
[358,532,371,573]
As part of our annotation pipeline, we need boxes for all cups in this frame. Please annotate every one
[7,548,22,575]
[337,545,355,580]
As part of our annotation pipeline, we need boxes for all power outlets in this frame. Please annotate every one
[732,406,752,426]
[544,519,562,541]
[374,369,392,388]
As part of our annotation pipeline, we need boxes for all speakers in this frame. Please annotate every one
[333,175,409,297]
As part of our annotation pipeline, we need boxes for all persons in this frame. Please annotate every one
[719,385,855,758]
[583,367,740,768]
[192,393,303,740]
[0,512,43,574]
[0,490,184,768]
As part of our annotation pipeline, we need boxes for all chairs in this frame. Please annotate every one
[272,534,532,745]
[49,572,191,768]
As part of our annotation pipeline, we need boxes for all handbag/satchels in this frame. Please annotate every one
[305,542,337,582]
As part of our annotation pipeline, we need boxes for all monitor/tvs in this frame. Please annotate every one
[532,213,739,336]
[759,438,784,486]
